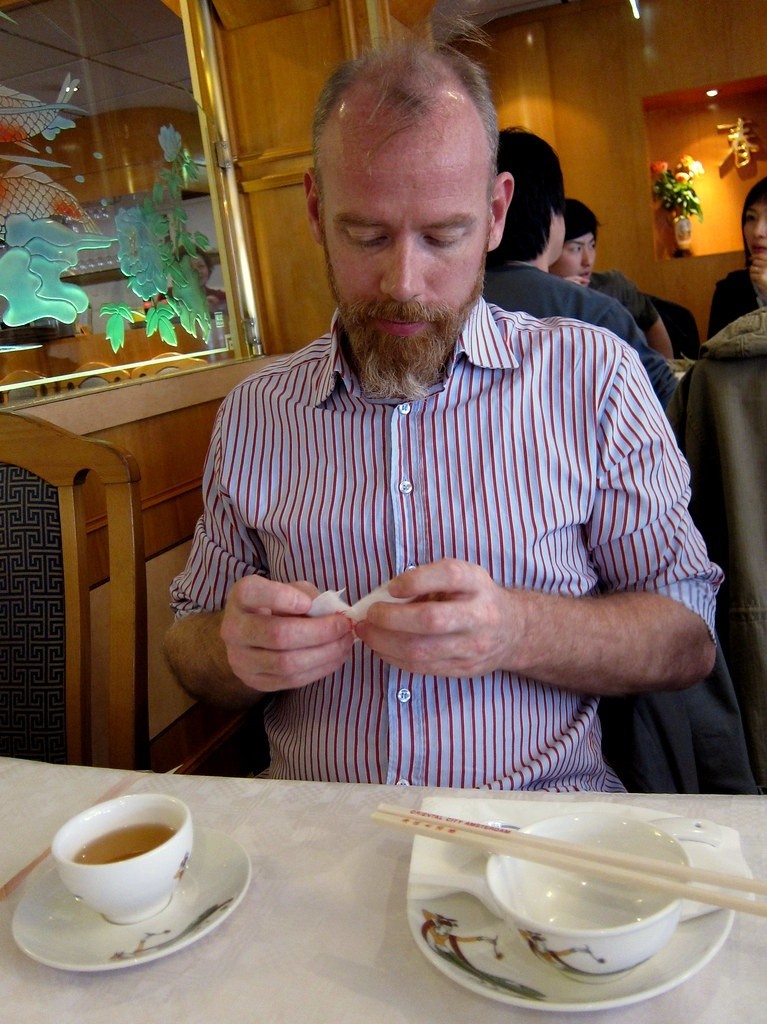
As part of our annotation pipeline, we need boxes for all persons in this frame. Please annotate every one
[483,124,681,410]
[162,43,727,794]
[549,198,673,359]
[707,176,767,340]
[664,307,767,795]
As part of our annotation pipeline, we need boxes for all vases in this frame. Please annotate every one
[674,216,692,250]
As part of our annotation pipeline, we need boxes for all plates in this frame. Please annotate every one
[11,826,253,971]
[407,802,734,1012]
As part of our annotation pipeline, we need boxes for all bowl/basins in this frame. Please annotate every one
[486,812,690,974]
[50,793,193,927]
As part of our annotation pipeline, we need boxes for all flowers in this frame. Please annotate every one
[651,155,705,223]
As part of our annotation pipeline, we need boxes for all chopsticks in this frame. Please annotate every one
[369,802,767,918]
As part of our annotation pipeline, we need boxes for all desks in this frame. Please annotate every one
[0,755,767,1024]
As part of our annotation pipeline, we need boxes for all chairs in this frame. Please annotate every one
[0,352,210,771]
[644,292,700,360]
